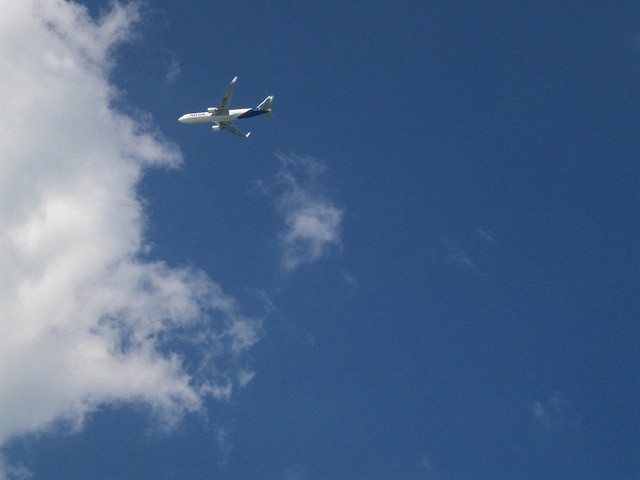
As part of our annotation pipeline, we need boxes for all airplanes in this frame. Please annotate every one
[177,75,273,139]
